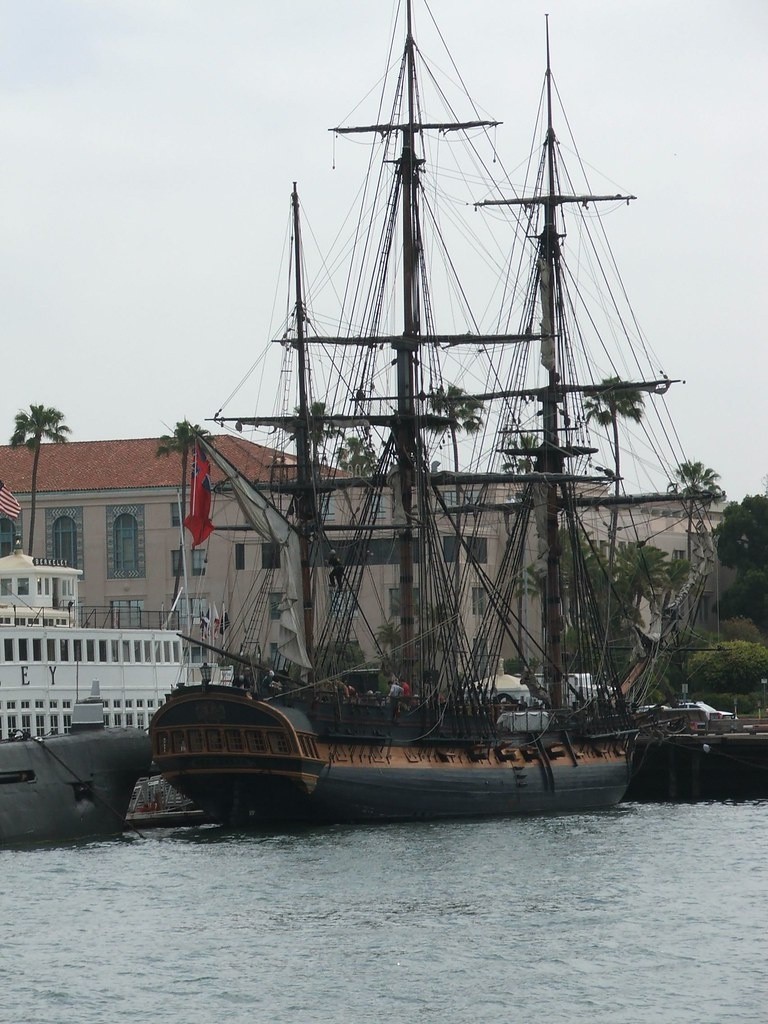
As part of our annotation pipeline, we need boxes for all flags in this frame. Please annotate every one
[213,607,220,638]
[0,481,21,518]
[200,609,210,641]
[220,607,230,635]
[183,436,214,548]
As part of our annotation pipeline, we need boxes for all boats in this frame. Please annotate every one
[0,678,164,844]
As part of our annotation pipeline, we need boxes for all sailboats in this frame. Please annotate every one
[150,0,723,836]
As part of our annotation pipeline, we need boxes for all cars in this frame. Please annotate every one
[675,700,733,721]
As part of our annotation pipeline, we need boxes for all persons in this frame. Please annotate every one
[486,696,493,706]
[434,688,446,714]
[325,549,344,589]
[517,696,527,711]
[714,713,724,735]
[234,667,286,696]
[338,679,418,712]
